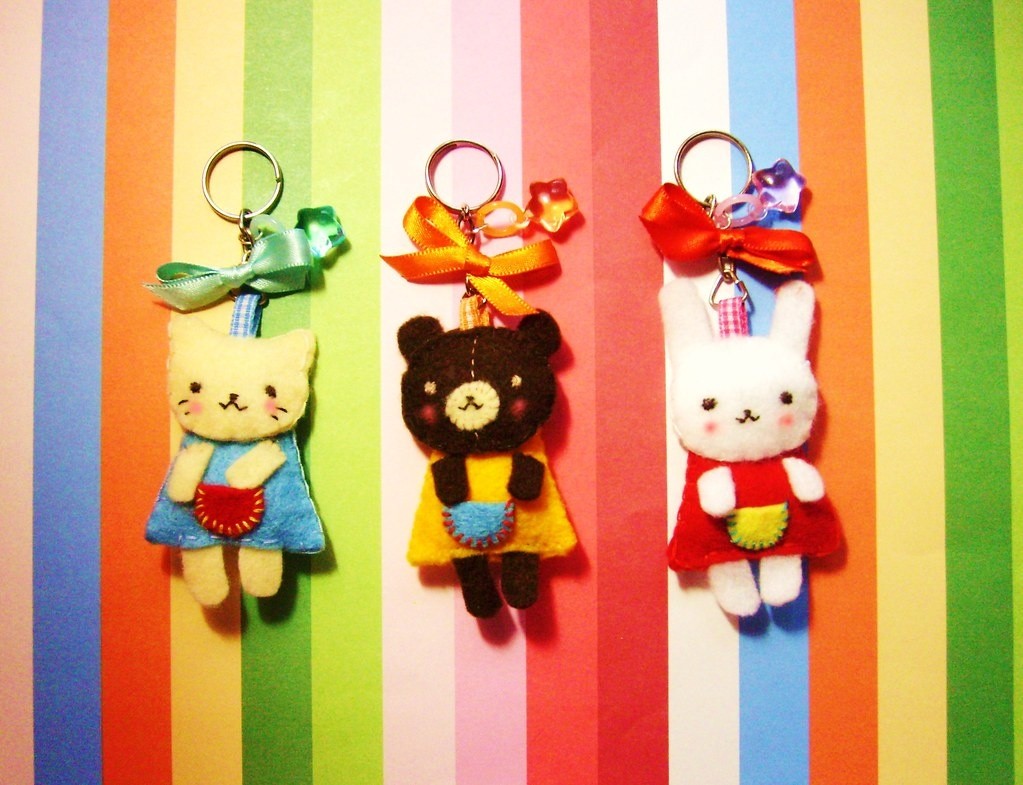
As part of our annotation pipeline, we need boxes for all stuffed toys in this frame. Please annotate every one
[655,275,844,621]
[144,312,327,609]
[396,308,580,619]
